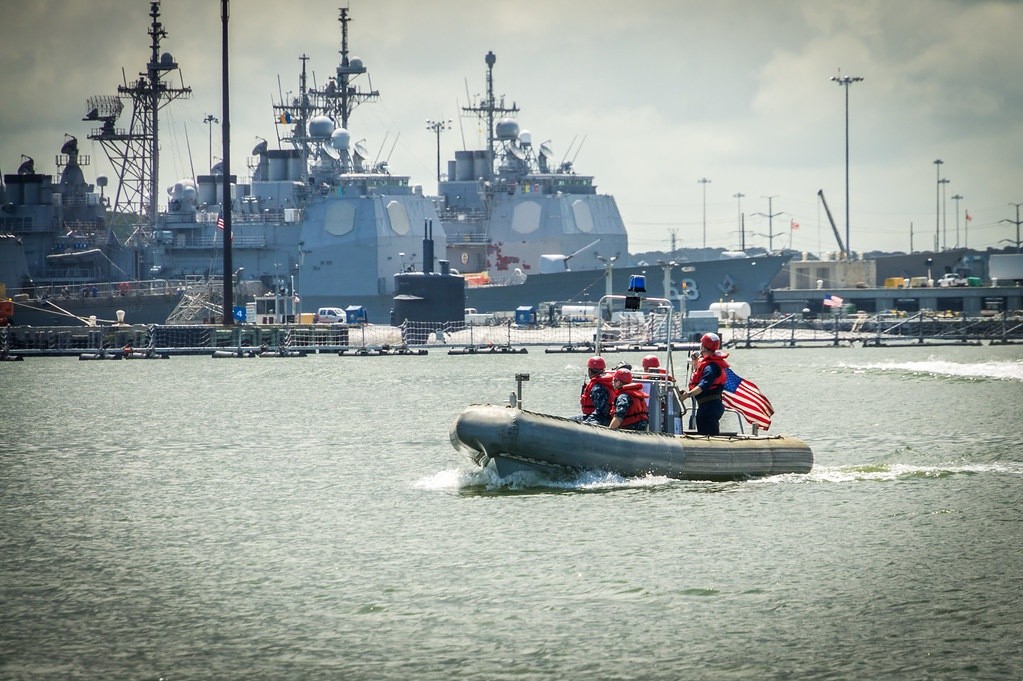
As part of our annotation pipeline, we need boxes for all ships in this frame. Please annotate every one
[0,0,970,324]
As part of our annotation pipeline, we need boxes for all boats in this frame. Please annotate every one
[450,273,814,484]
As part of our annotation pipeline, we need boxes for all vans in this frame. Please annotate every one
[317,308,346,323]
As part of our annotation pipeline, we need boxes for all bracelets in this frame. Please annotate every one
[688,392,694,398]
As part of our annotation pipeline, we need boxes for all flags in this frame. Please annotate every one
[792,222,801,229]
[217,209,233,241]
[722,367,774,431]
[824,292,843,307]
[965,212,972,221]
[278,111,293,124]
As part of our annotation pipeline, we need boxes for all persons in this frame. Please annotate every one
[42,283,130,299]
[313,314,320,324]
[581,355,675,432]
[680,333,730,437]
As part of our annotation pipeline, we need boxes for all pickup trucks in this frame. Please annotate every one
[937,274,969,287]
[464,308,495,326]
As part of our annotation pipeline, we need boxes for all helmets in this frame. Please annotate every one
[588,356,606,369]
[613,367,632,383]
[642,355,659,368]
[700,333,720,350]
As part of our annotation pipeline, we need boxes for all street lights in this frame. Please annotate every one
[831,75,861,261]
[697,178,711,261]
[732,192,745,249]
